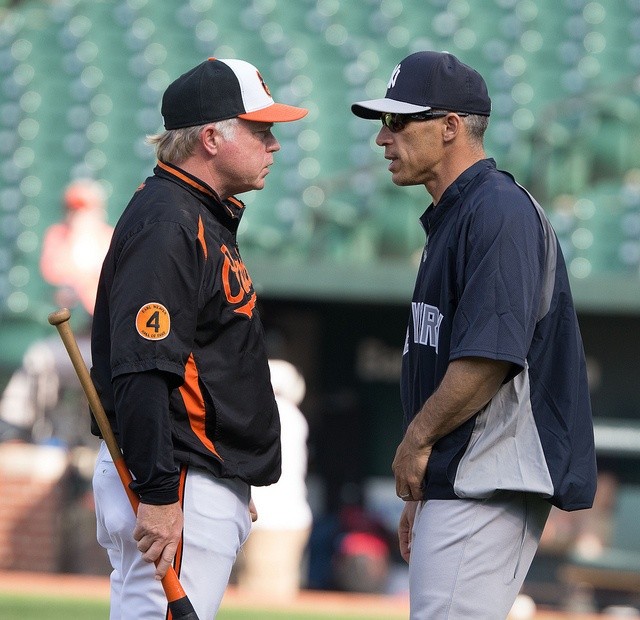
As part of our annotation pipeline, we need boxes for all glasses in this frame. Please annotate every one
[379,110,469,133]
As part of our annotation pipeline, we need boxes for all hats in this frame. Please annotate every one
[160,57,309,130]
[350,50,492,119]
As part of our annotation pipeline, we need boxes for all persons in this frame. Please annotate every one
[38,178,124,321]
[85,56,310,619]
[350,49,598,619]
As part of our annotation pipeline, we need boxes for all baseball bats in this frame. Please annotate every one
[47,308,200,620]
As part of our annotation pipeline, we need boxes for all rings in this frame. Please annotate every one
[398,492,413,500]
